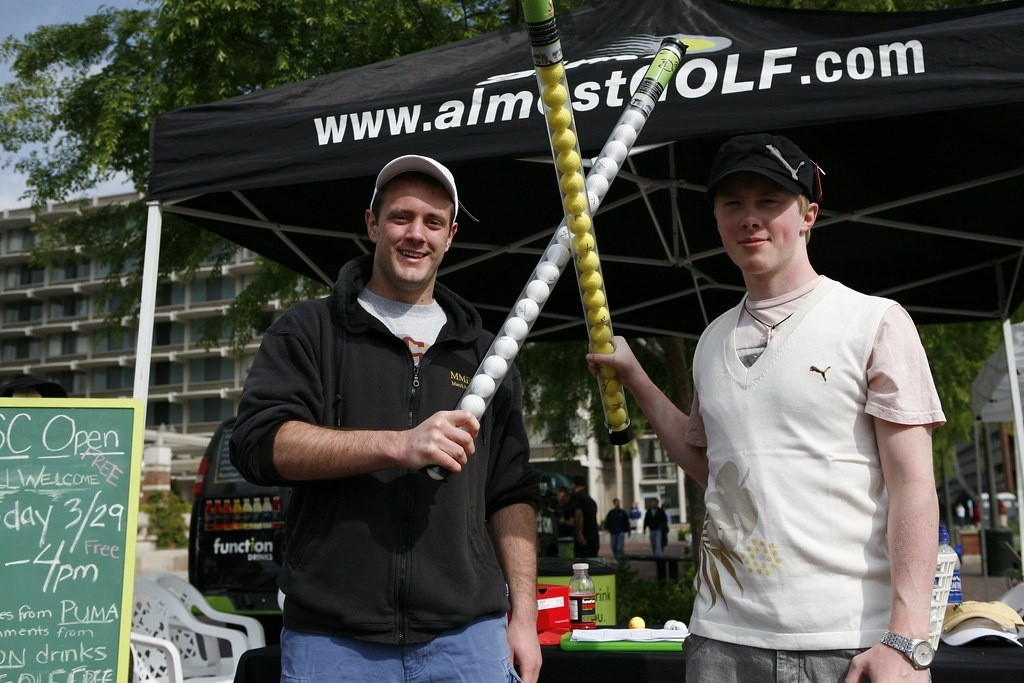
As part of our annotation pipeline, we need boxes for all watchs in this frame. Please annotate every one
[880,629,938,670]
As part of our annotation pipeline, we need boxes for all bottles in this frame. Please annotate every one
[933,524,963,606]
[569,563,595,629]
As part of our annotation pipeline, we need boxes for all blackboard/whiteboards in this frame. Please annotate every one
[0,398,147,683]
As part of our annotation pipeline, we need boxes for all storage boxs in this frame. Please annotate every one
[535,556,617,626]
[507,583,572,632]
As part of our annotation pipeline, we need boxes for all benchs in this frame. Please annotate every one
[626,553,692,588]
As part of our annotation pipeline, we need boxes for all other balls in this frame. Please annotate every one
[538,63,631,431]
[628,615,646,629]
[443,36,689,466]
[664,620,687,633]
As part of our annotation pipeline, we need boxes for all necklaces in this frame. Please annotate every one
[743,300,794,345]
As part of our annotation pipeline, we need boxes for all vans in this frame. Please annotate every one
[187,416,286,622]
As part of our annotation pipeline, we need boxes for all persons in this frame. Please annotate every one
[585,135,947,682]
[232,155,539,683]
[538,477,669,579]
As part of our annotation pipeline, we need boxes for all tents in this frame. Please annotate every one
[135,1,1023,466]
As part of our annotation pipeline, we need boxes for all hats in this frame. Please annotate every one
[940,601,1023,648]
[370,155,479,225]
[707,134,825,209]
[573,475,586,486]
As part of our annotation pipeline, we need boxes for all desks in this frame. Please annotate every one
[542,624,1024,683]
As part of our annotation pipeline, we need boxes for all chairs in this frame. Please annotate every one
[129,572,266,683]
